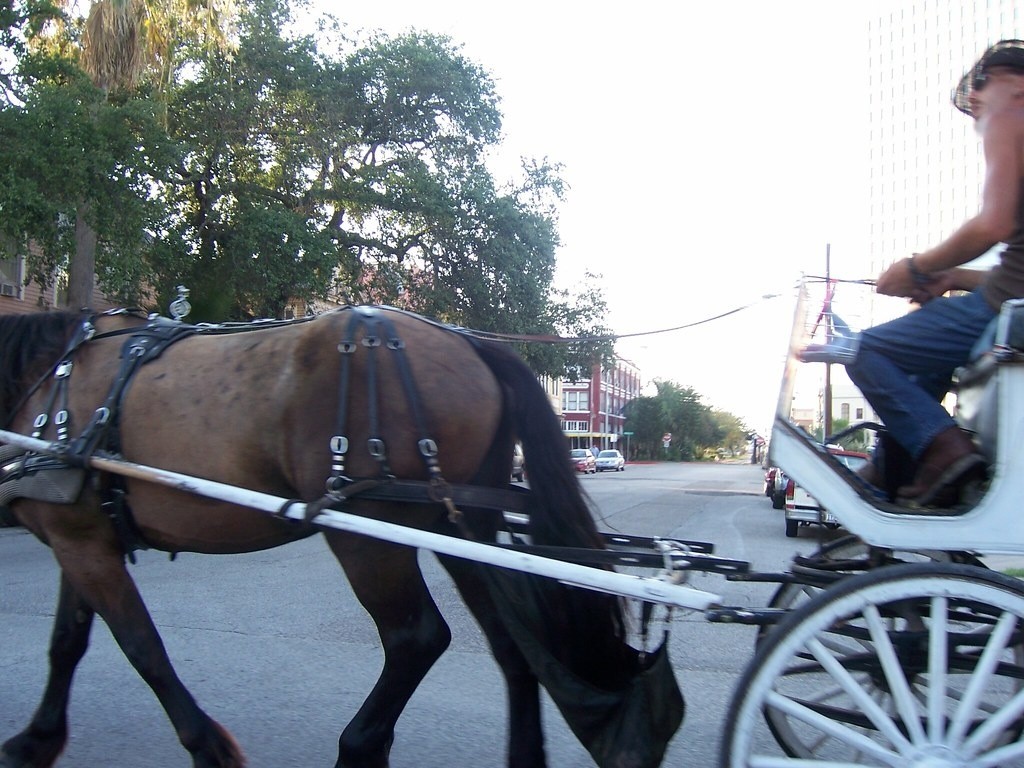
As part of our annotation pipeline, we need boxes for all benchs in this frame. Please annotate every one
[945,299,1024,386]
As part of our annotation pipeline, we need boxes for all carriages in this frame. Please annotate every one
[0,284,1024,768]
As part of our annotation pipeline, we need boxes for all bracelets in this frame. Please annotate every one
[906,253,931,283]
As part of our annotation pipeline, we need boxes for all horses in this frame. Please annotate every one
[0,307,639,768]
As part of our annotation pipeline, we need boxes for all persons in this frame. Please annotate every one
[590,444,600,458]
[845,38,1024,507]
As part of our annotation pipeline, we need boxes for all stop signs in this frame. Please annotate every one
[662,433,672,442]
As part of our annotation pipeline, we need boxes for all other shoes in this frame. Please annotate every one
[853,461,914,485]
[895,425,993,508]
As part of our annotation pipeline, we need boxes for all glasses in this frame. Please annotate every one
[970,69,1008,91]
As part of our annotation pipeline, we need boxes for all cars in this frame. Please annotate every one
[509,443,524,483]
[569,448,597,474]
[595,449,625,472]
[763,443,873,537]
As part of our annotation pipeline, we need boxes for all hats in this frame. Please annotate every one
[953,38,1024,120]
